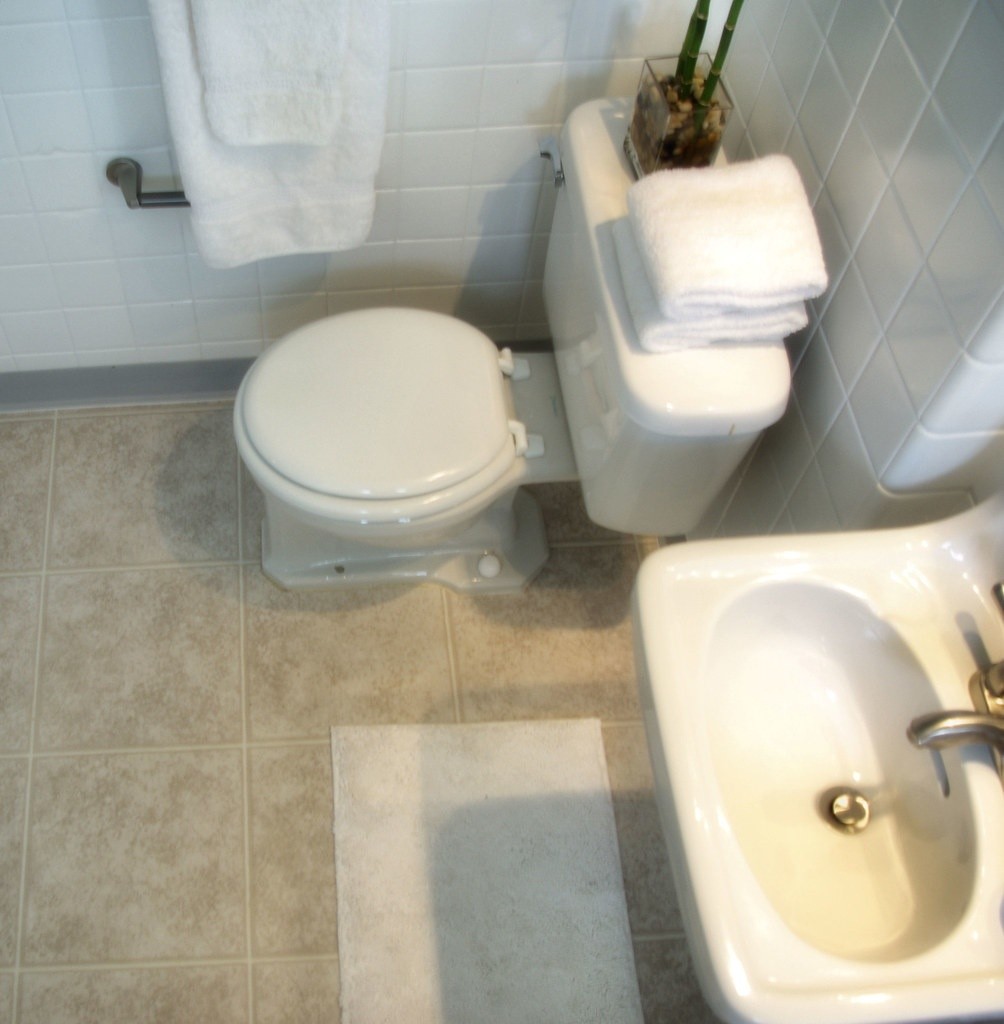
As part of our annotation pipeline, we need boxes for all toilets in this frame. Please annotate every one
[229,96,793,597]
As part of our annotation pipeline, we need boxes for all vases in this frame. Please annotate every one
[620,52,737,175]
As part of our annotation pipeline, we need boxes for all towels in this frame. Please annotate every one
[611,154,829,353]
[187,1,348,150]
[329,717,646,1023]
[149,0,390,273]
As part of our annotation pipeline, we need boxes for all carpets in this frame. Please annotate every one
[329,717,651,1024]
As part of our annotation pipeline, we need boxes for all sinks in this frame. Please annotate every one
[633,490,1004,1024]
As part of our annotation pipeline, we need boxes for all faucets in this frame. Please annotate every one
[906,660,1003,788]
